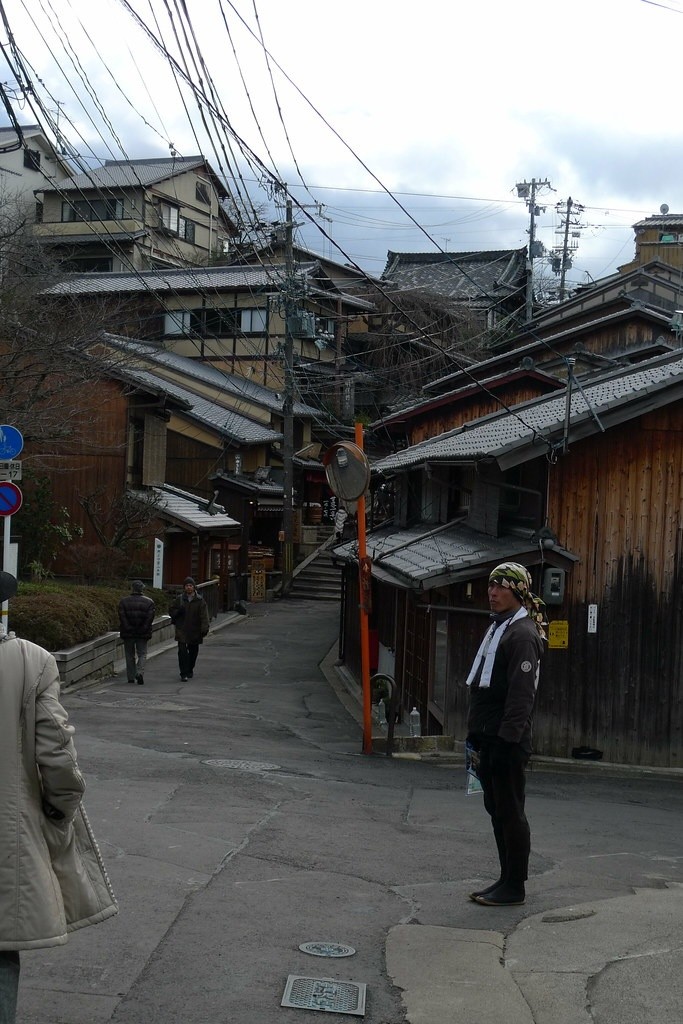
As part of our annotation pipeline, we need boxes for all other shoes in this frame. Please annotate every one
[128,680,134,683]
[181,674,188,682]
[468,877,506,900]
[187,673,193,678]
[135,673,144,684]
[475,881,526,905]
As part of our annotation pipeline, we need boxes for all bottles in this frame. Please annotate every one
[410,707,421,737]
[379,699,385,722]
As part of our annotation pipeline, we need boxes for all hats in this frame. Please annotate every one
[0,571,19,602]
[183,577,196,587]
[131,580,145,594]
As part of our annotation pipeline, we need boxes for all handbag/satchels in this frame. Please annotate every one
[169,594,185,625]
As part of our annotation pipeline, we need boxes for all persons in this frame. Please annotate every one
[117,580,157,685]
[0,572,119,1024]
[169,577,210,681]
[469,562,550,905]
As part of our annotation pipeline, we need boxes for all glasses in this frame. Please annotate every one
[486,583,509,592]
